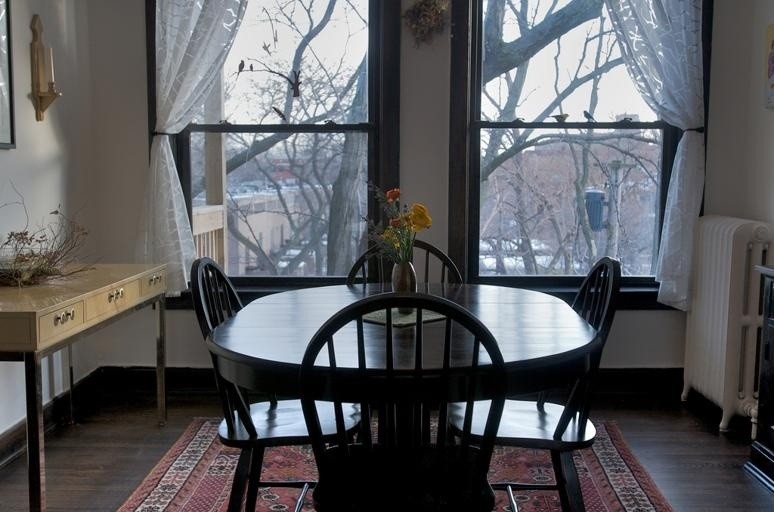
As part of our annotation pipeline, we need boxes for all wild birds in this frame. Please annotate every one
[583,110,598,122]
[235,60,244,80]
[546,114,570,122]
[249,64,253,71]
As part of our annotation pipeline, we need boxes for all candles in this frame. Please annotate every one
[43,39,56,83]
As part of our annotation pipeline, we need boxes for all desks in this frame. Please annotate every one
[201,283,600,403]
[0,261,168,512]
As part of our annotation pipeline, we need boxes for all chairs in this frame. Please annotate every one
[189,258,366,511]
[346,233,466,286]
[296,291,509,510]
[442,257,622,511]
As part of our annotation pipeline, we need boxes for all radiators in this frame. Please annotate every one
[679,213,771,446]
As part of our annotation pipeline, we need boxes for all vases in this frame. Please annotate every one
[392,257,418,315]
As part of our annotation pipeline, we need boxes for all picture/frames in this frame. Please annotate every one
[2,0,17,153]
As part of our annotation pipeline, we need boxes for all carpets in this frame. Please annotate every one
[114,415,675,511]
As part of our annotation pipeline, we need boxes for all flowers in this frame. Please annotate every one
[403,0,452,43]
[360,180,433,263]
